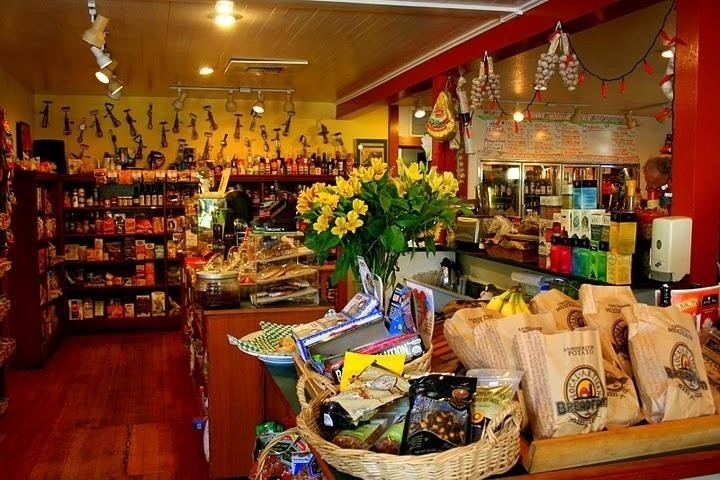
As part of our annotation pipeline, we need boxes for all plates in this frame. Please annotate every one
[236,329,296,364]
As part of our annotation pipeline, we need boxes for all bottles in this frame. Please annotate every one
[481,170,553,217]
[192,270,242,311]
[63,183,165,234]
[230,149,360,204]
[624,179,638,215]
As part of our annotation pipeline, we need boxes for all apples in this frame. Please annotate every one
[452,388,469,399]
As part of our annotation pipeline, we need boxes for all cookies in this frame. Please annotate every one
[409,408,465,444]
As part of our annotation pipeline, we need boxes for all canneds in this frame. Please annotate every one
[117,193,164,207]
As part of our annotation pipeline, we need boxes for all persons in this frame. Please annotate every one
[643,157,672,199]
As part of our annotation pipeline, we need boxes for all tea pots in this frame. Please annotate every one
[436,256,463,295]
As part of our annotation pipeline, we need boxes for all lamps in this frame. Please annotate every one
[169,87,297,113]
[413,97,426,118]
[81,0,127,100]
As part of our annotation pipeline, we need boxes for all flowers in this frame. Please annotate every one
[297,156,469,304]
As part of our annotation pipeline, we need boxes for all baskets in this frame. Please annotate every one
[293,343,434,398]
[295,371,523,480]
[255,427,301,480]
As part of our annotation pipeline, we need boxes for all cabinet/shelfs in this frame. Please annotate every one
[481,159,641,219]
